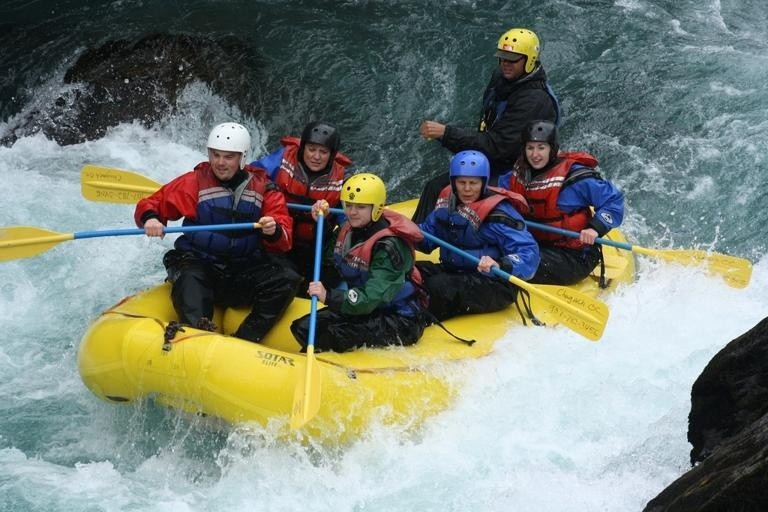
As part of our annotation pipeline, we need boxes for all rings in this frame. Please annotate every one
[482,261,485,263]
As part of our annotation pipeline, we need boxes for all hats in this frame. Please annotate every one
[493,48,523,62]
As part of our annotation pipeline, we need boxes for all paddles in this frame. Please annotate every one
[422,231,608,341]
[1,222,260,260]
[291,202,325,431]
[80,165,344,214]
[528,221,752,288]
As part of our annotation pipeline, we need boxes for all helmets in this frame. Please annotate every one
[205,122,252,171]
[447,150,491,195]
[300,120,340,173]
[338,172,387,222]
[520,119,560,165]
[498,28,540,74]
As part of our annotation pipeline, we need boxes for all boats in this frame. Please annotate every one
[75,197,642,466]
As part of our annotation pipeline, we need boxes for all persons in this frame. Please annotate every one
[492,119,624,286]
[285,169,432,354]
[412,148,542,326]
[243,118,355,298]
[404,27,562,221]
[132,118,302,342]
